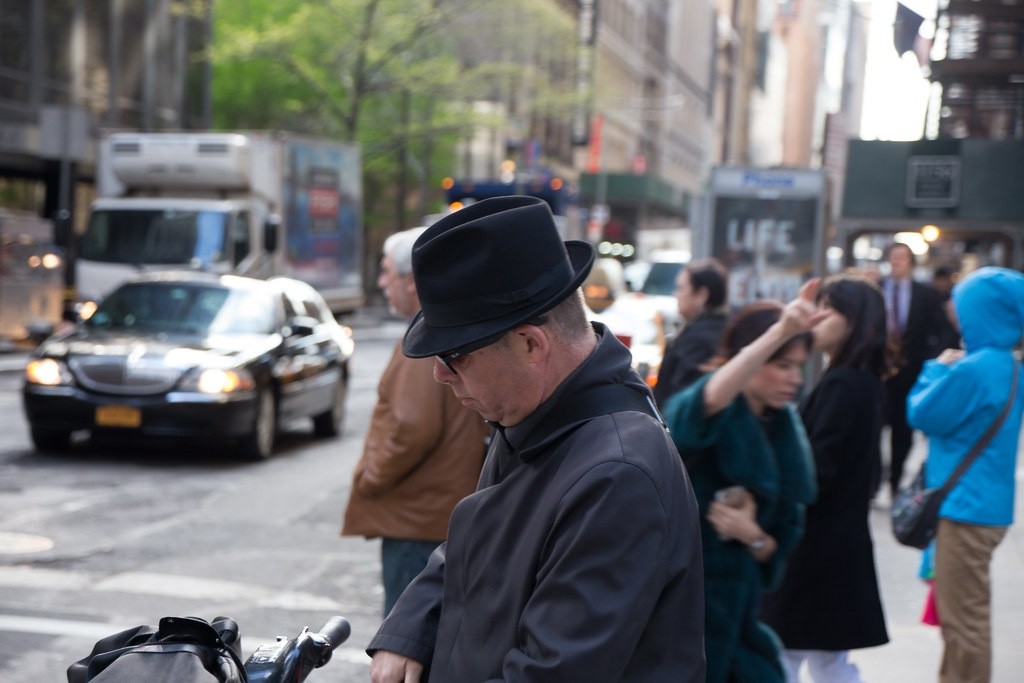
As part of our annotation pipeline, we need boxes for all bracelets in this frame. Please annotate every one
[751,538,766,548]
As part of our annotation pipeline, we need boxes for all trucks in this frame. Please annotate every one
[72,129,366,318]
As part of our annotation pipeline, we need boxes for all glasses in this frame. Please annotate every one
[434,316,549,375]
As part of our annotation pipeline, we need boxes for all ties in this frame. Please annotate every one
[892,284,900,332]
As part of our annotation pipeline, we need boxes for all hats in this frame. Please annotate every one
[403,195,598,357]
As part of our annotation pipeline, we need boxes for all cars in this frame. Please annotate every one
[18,268,355,460]
[580,248,691,384]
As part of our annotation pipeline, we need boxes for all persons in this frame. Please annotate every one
[655,242,1024,683]
[364,195,706,683]
[341,226,491,619]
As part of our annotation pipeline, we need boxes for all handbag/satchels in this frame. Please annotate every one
[892,468,941,547]
